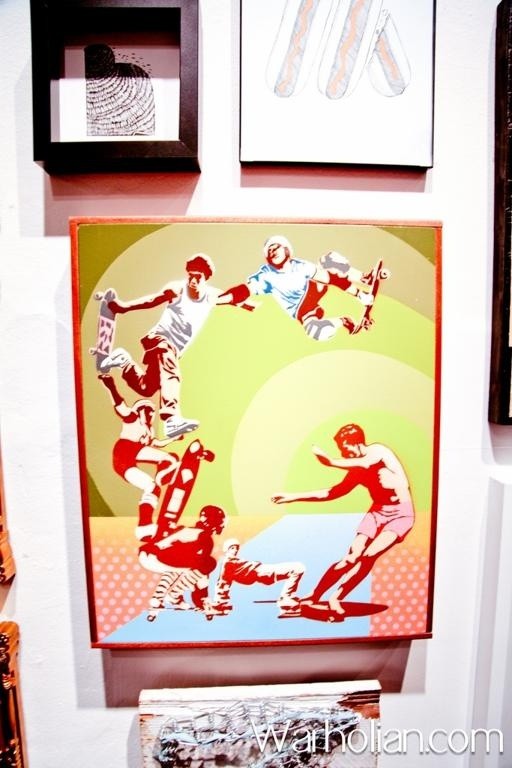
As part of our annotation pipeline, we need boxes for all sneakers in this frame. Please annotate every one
[172,599,196,610]
[328,596,347,616]
[163,414,201,438]
[340,316,356,335]
[134,522,158,542]
[147,598,166,610]
[359,267,375,286]
[155,461,181,487]
[277,596,318,611]
[100,346,132,371]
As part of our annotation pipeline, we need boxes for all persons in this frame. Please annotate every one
[274,423,417,622]
[137,508,227,611]
[99,374,184,544]
[219,237,376,342]
[106,256,257,435]
[218,541,305,614]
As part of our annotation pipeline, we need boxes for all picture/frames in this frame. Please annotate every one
[66,215,448,653]
[28,0,203,178]
[0,617,35,768]
[486,1,511,426]
[239,0,436,171]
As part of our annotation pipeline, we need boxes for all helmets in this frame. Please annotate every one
[198,504,226,529]
[130,398,156,414]
[263,234,294,260]
[222,538,240,554]
